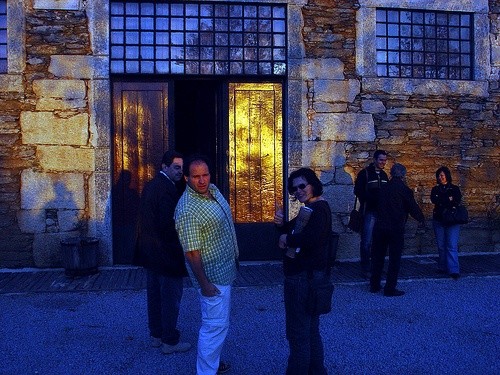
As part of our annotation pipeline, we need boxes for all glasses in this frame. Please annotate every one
[291,183,309,191]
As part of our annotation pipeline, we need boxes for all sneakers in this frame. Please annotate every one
[151,336,192,353]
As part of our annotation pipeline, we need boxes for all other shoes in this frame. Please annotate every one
[370,285,382,293]
[383,286,405,297]
[216,362,232,373]
[437,269,461,279]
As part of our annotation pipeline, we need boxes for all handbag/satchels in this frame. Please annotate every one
[433,199,469,224]
[348,209,361,233]
[315,231,341,314]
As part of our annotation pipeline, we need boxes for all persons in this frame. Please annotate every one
[368,163,425,297]
[130,150,192,353]
[274,167,333,375]
[353,149,389,279]
[430,166,462,278]
[174,155,240,375]
